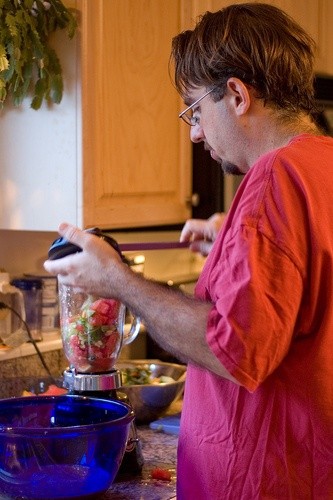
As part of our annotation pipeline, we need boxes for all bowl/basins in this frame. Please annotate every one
[118,359,189,423]
[0,395,134,500]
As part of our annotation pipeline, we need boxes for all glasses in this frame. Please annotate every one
[178,86,215,127]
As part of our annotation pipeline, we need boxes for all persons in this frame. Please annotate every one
[43,3,333,500]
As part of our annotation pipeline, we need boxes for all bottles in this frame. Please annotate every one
[9,277,46,343]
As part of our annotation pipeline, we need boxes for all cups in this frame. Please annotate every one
[14,277,44,340]
[1,272,24,345]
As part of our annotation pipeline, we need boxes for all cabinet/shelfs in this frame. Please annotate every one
[0,1,333,231]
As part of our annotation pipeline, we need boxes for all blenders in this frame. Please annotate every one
[47,227,145,483]
